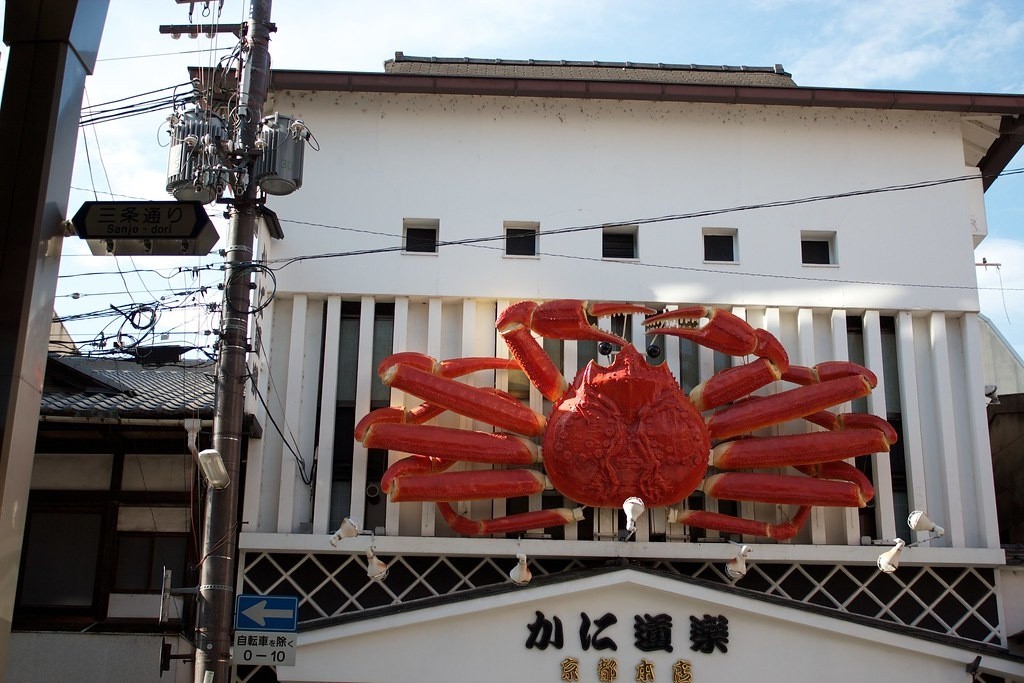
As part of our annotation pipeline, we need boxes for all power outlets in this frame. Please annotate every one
[876,539,905,574]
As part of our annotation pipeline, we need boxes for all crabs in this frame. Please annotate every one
[355,299,898,540]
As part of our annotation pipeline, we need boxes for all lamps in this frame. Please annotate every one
[725,545,752,581]
[364,548,389,582]
[327,517,360,548]
[623,497,645,532]
[907,510,945,537]
[985,384,1001,405]
[509,553,532,586]
